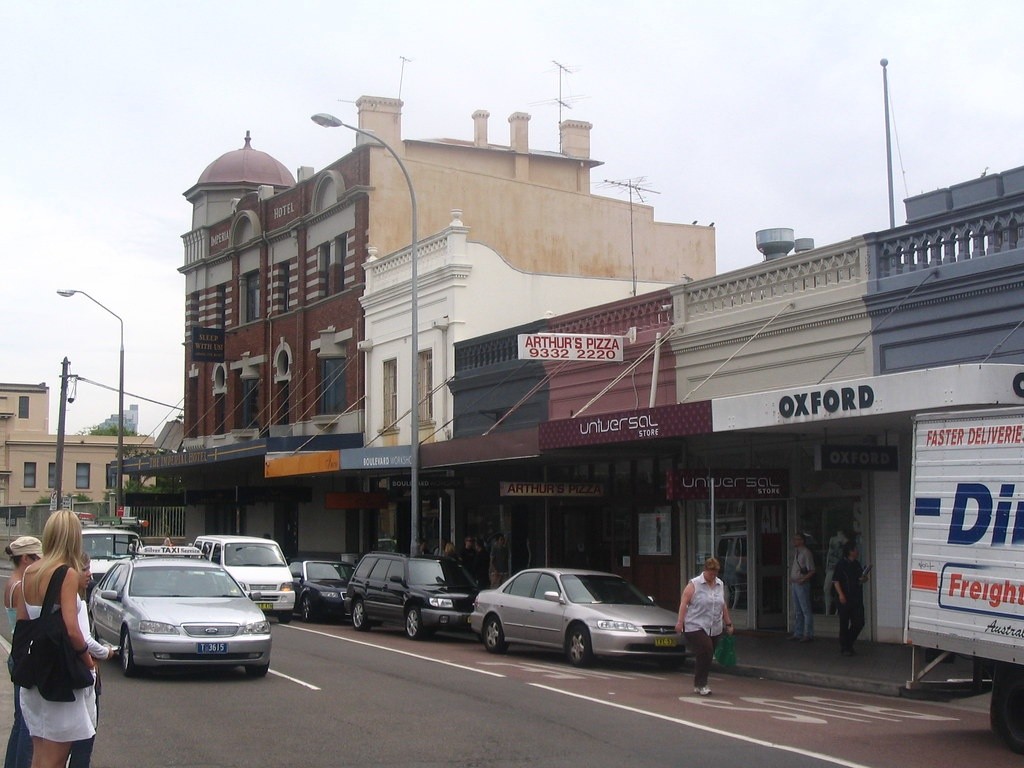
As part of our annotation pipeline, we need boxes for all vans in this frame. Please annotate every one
[193,534,296,622]
[78,526,145,589]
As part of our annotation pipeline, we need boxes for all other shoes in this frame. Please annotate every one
[841,648,854,656]
[800,636,812,643]
[787,636,798,641]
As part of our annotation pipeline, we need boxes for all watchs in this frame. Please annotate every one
[726,623,734,627]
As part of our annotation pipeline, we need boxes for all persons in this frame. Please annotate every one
[787,533,817,642]
[824,530,847,616]
[419,534,509,590]
[676,559,734,697]
[0,534,118,768]
[19,509,99,768]
[832,540,869,657]
[163,530,175,546]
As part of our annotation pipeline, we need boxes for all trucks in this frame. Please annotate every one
[903,405,1024,751]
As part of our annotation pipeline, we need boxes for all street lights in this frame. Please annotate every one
[313,114,418,558]
[57,290,125,520]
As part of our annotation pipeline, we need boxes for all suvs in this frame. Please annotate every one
[345,550,482,641]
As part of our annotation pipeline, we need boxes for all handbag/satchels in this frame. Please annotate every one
[713,631,742,667]
[10,564,95,702]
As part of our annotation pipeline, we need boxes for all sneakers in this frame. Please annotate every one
[694,686,712,696]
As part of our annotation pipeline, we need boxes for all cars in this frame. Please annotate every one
[90,546,272,680]
[470,567,688,668]
[289,557,353,622]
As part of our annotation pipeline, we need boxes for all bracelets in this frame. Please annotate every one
[76,643,89,656]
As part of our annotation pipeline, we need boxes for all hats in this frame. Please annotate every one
[9,535,44,560]
